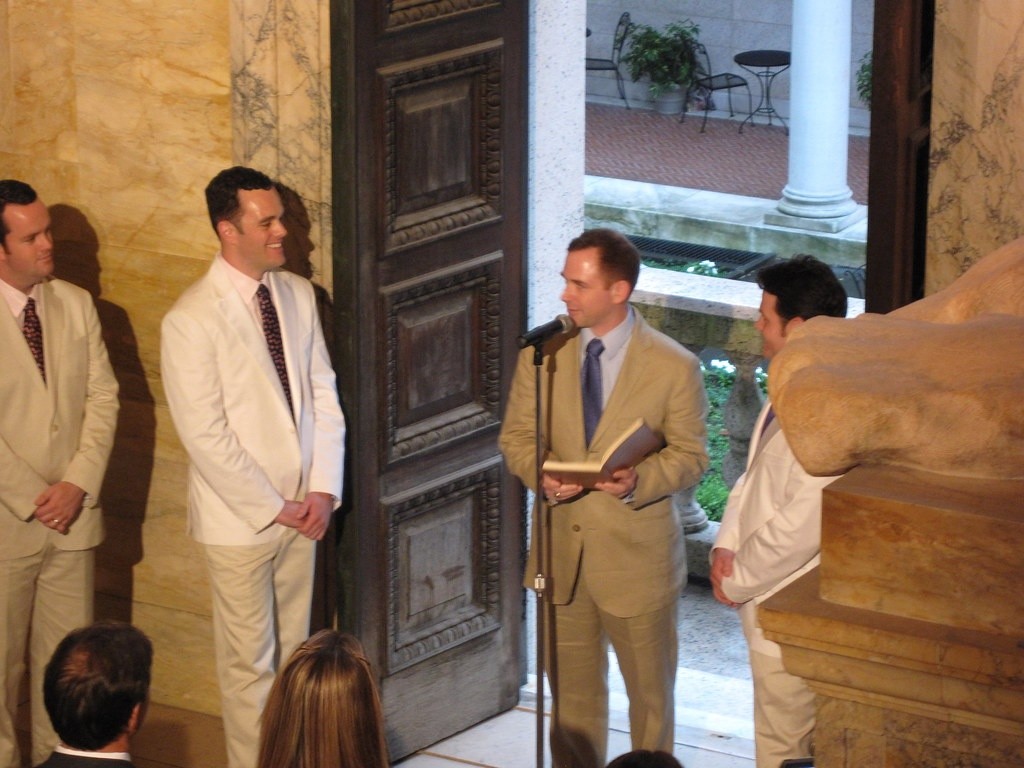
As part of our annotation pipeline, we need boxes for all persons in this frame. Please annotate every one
[0,178,118,768]
[257,631,392,768]
[707,254,849,768]
[159,167,346,768]
[497,228,707,768]
[36,620,153,768]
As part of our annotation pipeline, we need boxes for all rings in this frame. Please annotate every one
[53,519,62,524]
[555,492,561,498]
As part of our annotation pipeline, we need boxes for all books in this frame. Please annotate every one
[543,416,659,488]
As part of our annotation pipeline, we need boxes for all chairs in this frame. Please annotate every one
[675,37,758,138]
[583,9,633,115]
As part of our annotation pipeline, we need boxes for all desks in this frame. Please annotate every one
[731,47,793,141]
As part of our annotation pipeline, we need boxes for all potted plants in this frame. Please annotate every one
[612,17,712,121]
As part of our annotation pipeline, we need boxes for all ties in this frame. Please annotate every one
[581,338,604,448]
[255,284,294,419]
[759,406,774,438]
[23,298,46,382]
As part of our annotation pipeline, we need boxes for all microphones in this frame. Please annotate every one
[516,315,574,350]
